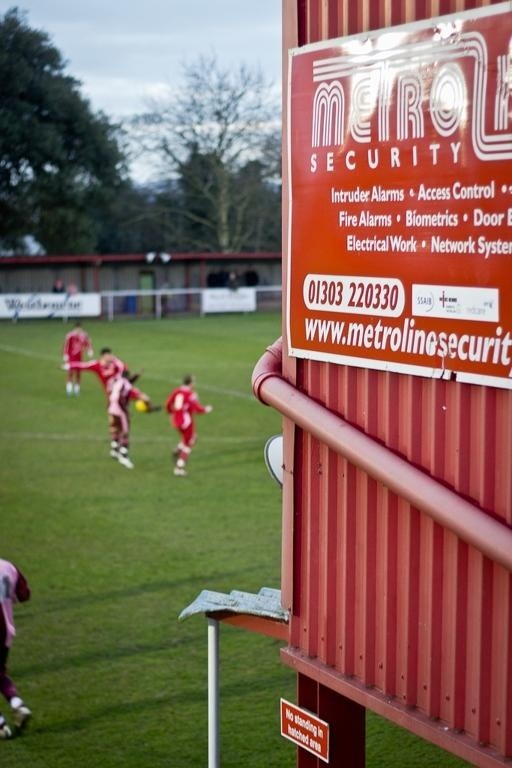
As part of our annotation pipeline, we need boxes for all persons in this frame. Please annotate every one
[61,319,95,393]
[1,554,32,740]
[106,367,151,469]
[57,348,163,414]
[164,374,213,476]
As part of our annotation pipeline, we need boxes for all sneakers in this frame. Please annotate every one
[0,705,31,740]
[66,382,81,396]
[173,468,187,477]
[109,450,134,470]
